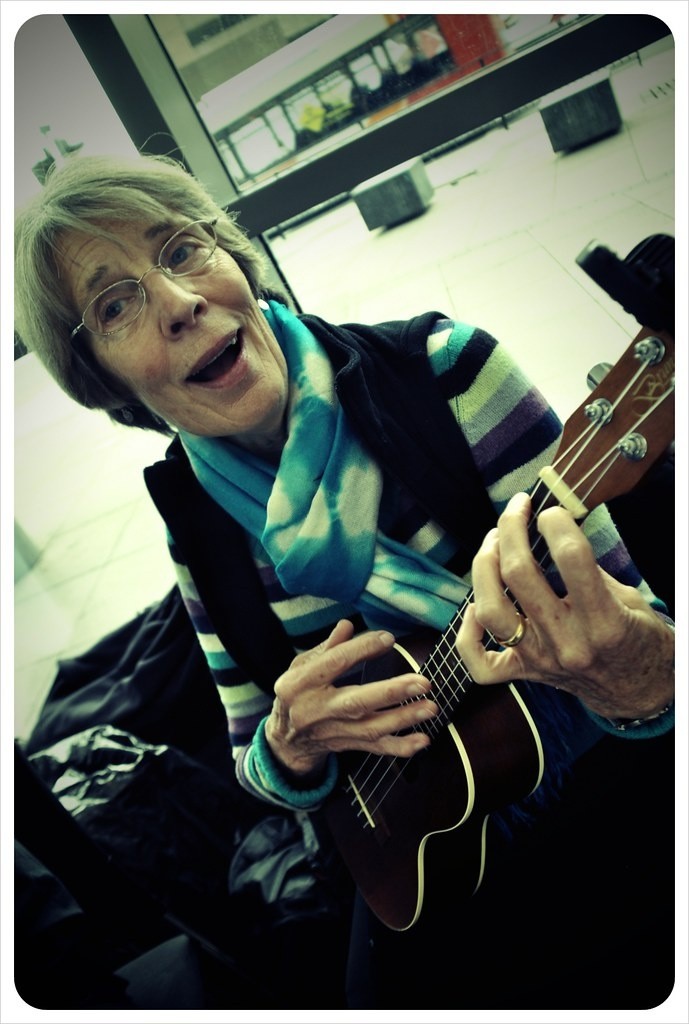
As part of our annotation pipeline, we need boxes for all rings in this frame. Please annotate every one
[498,612,527,647]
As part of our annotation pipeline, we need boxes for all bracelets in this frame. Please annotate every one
[608,702,672,731]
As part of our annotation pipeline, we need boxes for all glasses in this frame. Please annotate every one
[70,216,220,336]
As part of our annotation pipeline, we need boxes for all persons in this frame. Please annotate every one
[16,154,675,1009]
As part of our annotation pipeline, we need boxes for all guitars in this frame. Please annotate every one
[318,327,674,934]
